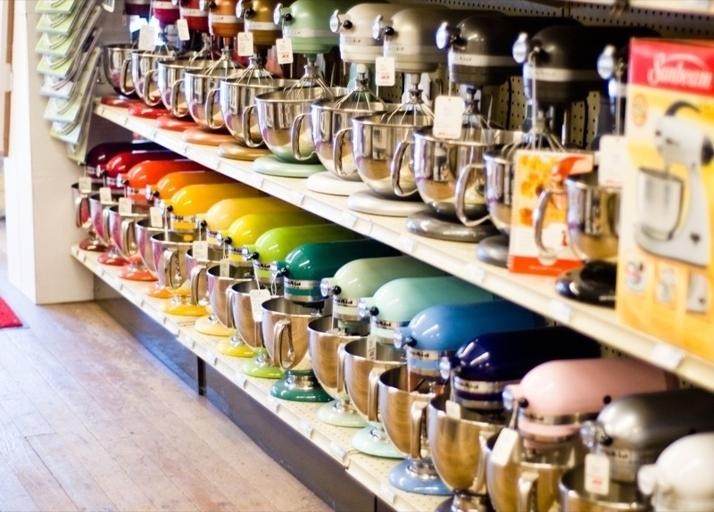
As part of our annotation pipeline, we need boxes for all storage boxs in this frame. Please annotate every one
[615,34,713,369]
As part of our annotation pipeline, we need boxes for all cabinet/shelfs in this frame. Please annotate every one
[56,0,713,512]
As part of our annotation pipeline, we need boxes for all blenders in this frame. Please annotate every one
[73,2,711,509]
